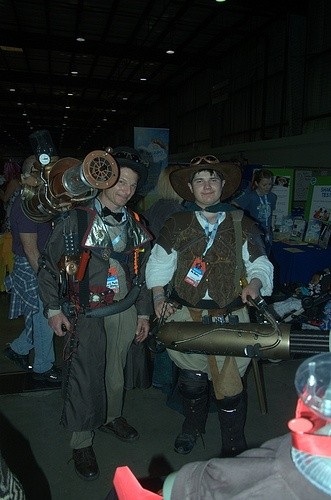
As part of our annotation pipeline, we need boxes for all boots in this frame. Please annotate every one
[172,369,210,454]
[214,390,246,457]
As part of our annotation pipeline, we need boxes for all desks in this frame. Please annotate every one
[269,242,331,283]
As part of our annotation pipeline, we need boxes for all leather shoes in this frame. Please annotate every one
[71,445,98,480]
[96,416,138,441]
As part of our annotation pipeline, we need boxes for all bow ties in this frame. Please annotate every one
[100,206,124,223]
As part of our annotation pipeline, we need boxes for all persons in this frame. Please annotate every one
[230,170,277,262]
[37,147,154,481]
[274,271,327,317]
[0,153,63,383]
[146,154,274,458]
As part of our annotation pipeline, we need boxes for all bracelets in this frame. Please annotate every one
[153,294,165,302]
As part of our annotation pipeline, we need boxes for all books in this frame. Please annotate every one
[272,215,331,253]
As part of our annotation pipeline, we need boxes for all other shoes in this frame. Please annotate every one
[33,366,62,382]
[2,345,32,370]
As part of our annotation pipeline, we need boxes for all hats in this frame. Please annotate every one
[110,146,147,187]
[168,154,241,201]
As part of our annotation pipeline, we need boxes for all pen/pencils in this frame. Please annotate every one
[320,225,327,236]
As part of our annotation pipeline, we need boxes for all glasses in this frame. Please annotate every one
[112,151,138,161]
[189,155,219,164]
